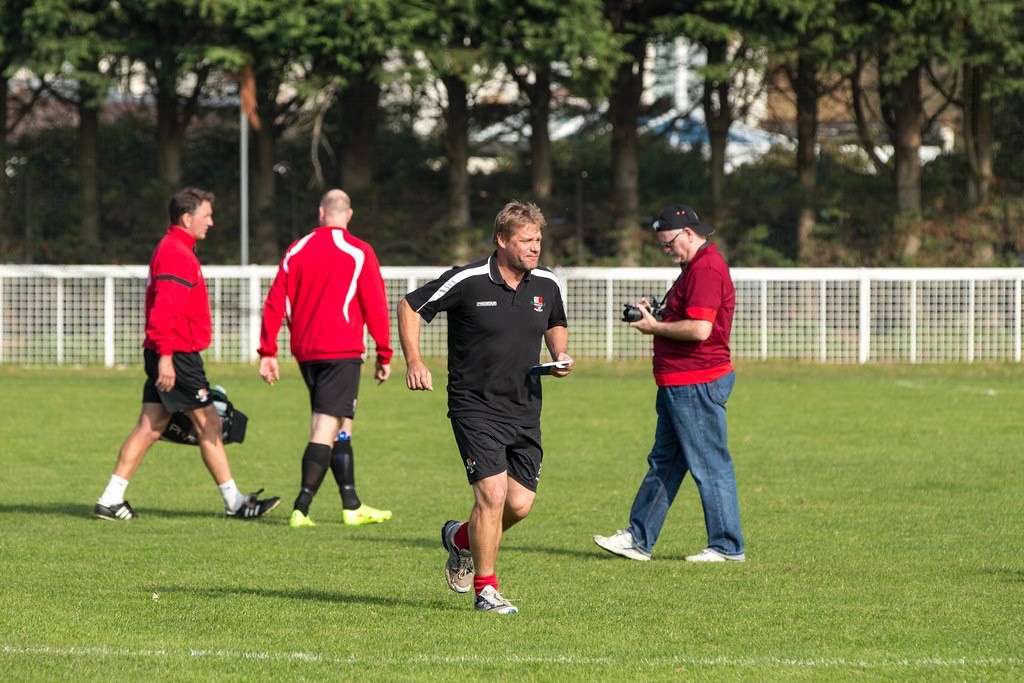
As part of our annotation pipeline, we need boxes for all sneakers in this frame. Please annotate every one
[473,584,519,616]
[684,548,745,564]
[224,487,281,521]
[592,529,651,561]
[341,502,393,526]
[93,497,145,523]
[289,509,317,528]
[441,519,476,593]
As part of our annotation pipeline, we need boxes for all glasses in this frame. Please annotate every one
[660,230,684,248]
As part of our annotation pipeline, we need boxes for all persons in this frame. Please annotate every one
[256,189,395,525]
[91,186,282,520]
[593,205,747,563]
[396,200,574,614]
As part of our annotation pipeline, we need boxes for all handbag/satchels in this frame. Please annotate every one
[157,386,247,446]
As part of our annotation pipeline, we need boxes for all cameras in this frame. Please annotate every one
[621,298,663,324]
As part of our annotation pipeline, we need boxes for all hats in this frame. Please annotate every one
[653,204,716,236]
